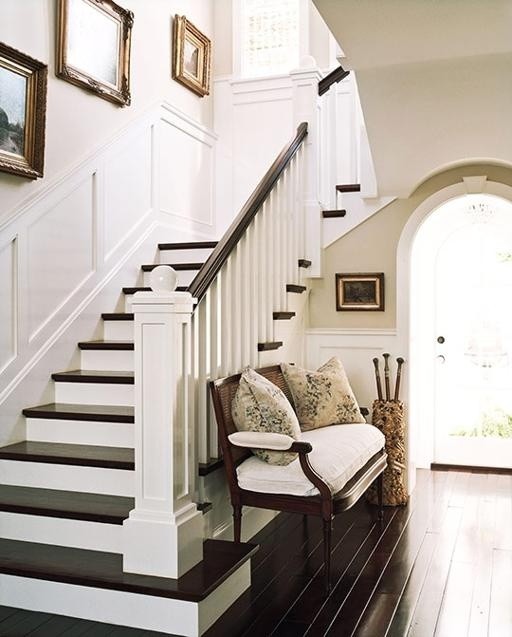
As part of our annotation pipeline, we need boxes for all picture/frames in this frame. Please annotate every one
[173,12,212,99]
[0,0,136,179]
[334,271,385,312]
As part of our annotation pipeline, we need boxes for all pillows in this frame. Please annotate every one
[230,356,367,468]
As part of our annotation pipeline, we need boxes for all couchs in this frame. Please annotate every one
[211,363,389,595]
